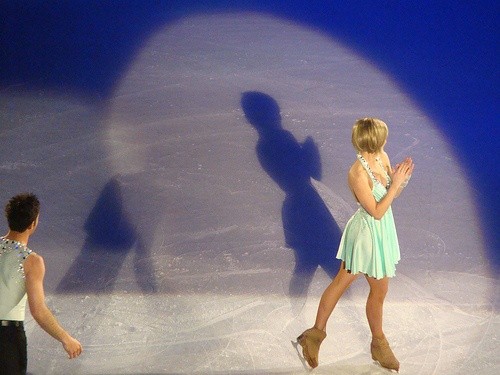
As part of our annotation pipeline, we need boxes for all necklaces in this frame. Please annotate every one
[376,156,381,167]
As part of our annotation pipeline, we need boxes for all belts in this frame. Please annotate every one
[1,320,24,327]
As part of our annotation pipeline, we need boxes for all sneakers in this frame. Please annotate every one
[291,326,327,372]
[370,336,401,374]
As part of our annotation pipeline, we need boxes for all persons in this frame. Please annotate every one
[0,193,83,375]
[291,118,414,374]
